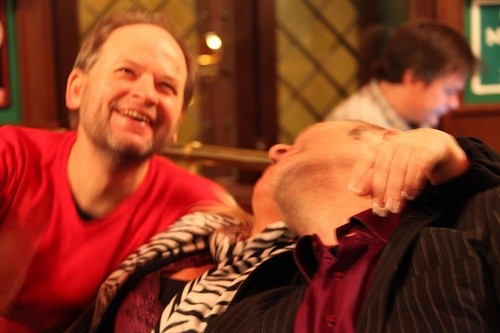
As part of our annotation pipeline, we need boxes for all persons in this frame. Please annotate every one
[325,19,478,131]
[93,118,500,333]
[0,12,240,333]
